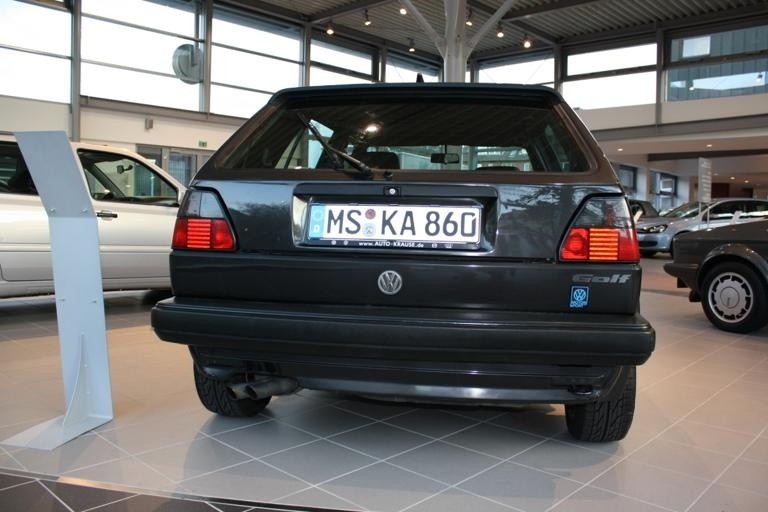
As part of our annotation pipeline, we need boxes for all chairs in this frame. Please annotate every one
[360,152,399,170]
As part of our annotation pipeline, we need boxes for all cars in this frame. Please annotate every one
[634,196,767,259]
[662,216,767,334]
[0,133,187,310]
[150,81,655,443]
